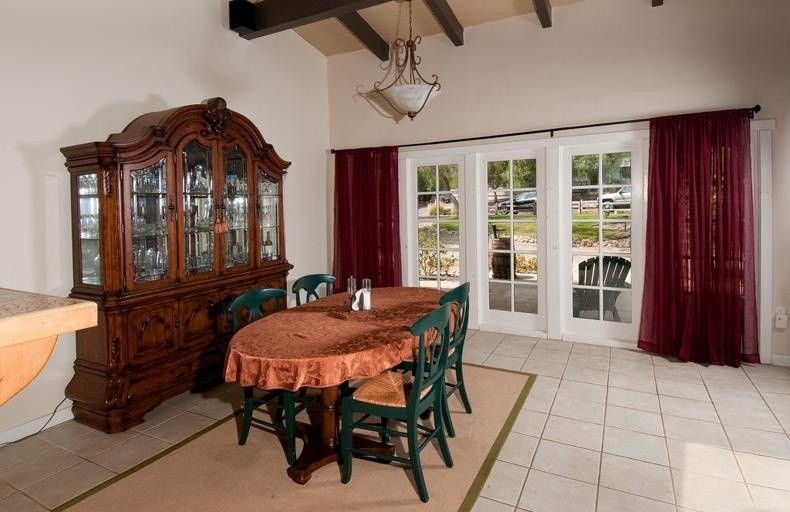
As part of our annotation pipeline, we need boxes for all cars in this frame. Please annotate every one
[428,191,451,204]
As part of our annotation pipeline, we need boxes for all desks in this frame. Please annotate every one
[222,285,455,483]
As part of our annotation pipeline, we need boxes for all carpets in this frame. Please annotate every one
[49,360,538,512]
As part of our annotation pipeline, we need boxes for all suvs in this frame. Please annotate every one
[496,191,536,215]
[597,184,631,212]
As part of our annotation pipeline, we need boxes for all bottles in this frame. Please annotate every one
[346,272,372,311]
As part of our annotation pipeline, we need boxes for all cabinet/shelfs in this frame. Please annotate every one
[65,265,295,435]
[59,109,178,282]
[180,96,255,282]
[256,124,289,273]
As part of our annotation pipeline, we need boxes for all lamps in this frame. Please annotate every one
[374,0,441,121]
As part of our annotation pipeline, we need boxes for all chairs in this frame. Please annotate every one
[337,302,458,502]
[572,255,633,322]
[389,281,473,420]
[292,274,337,308]
[229,288,307,466]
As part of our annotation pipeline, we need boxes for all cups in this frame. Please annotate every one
[76,162,276,284]
